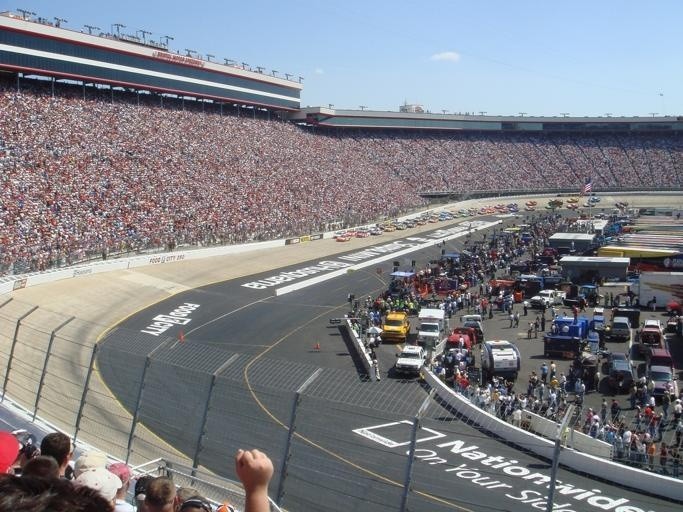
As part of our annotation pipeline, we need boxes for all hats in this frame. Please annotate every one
[0,430,234,512]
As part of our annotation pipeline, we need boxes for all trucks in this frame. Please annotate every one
[416,308,445,346]
[543,315,589,360]
[480,340,521,373]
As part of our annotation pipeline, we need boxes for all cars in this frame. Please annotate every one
[525,201,537,211]
[334,204,519,242]
[566,198,579,209]
[616,202,629,206]
[395,345,426,375]
[441,314,484,367]
[583,196,600,207]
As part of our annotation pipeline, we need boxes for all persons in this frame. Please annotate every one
[0,69,347,280]
[0,427,275,512]
[349,227,682,477]
[345,131,683,232]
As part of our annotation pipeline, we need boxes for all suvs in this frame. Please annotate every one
[610,316,675,396]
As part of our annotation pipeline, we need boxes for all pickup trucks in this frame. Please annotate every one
[531,289,566,309]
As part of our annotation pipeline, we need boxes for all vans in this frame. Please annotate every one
[383,312,410,342]
